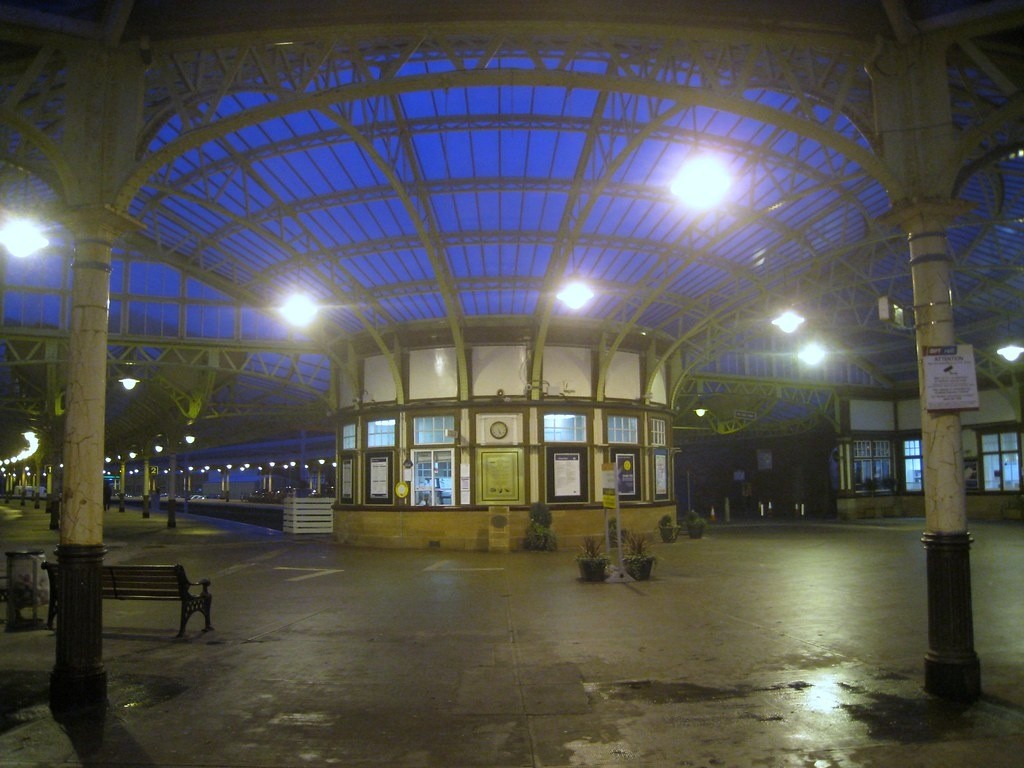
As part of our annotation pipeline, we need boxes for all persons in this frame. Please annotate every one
[103,482,112,512]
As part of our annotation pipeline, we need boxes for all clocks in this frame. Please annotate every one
[490,421,509,439]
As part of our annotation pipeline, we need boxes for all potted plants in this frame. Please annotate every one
[685,511,710,539]
[572,537,612,582]
[835,487,858,511]
[621,531,656,582]
[657,516,674,542]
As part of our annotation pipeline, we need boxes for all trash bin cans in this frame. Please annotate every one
[4,549,52,630]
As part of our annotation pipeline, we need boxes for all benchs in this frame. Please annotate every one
[39,561,211,644]
